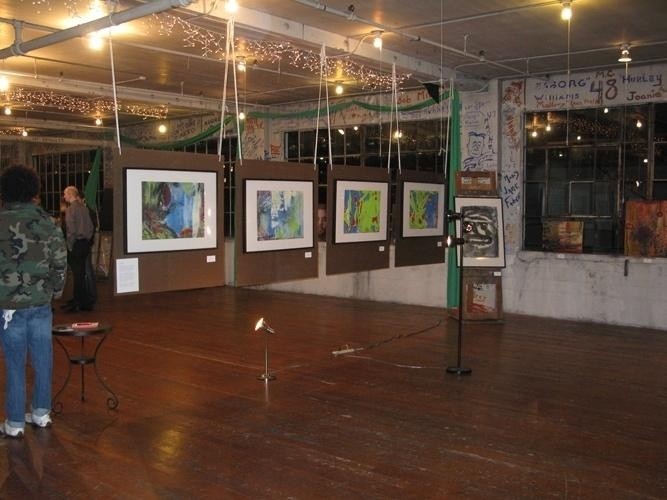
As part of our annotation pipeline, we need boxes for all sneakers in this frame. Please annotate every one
[25,413,52,428]
[61,297,96,311]
[0,418,25,438]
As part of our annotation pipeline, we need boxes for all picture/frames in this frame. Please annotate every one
[402,181,446,238]
[453,197,506,268]
[242,177,314,253]
[123,166,220,254]
[334,180,390,244]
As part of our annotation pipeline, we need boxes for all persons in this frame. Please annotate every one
[0,161,66,438]
[60,187,99,311]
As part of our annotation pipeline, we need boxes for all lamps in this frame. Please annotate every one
[253,318,277,381]
[444,232,473,377]
[617,41,632,63]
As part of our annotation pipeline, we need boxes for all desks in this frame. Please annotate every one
[50,324,119,417]
